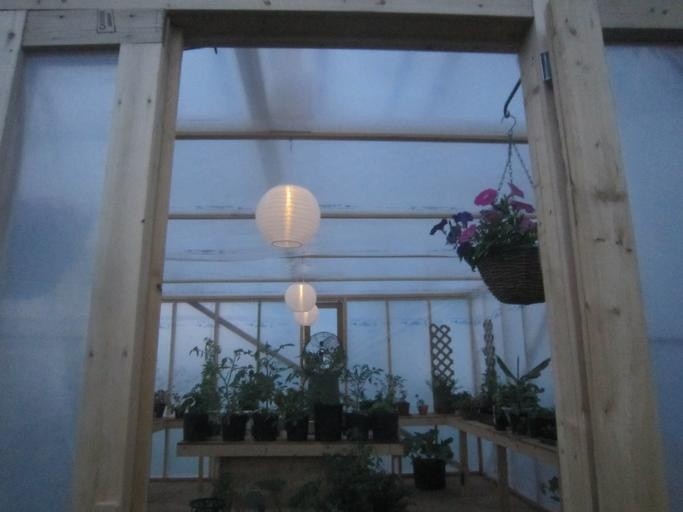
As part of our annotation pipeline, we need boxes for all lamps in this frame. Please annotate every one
[254,136,321,326]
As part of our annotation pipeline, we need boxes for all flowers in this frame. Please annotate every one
[429,182,538,272]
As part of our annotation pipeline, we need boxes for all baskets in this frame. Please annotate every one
[476,246,545,305]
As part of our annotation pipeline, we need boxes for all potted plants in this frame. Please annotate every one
[250,339,282,440]
[201,441,410,511]
[360,375,402,442]
[280,389,309,442]
[402,426,455,489]
[431,355,559,443]
[155,390,172,418]
[305,344,349,440]
[174,338,224,441]
[360,396,410,416]
[219,341,253,440]
[416,394,429,414]
